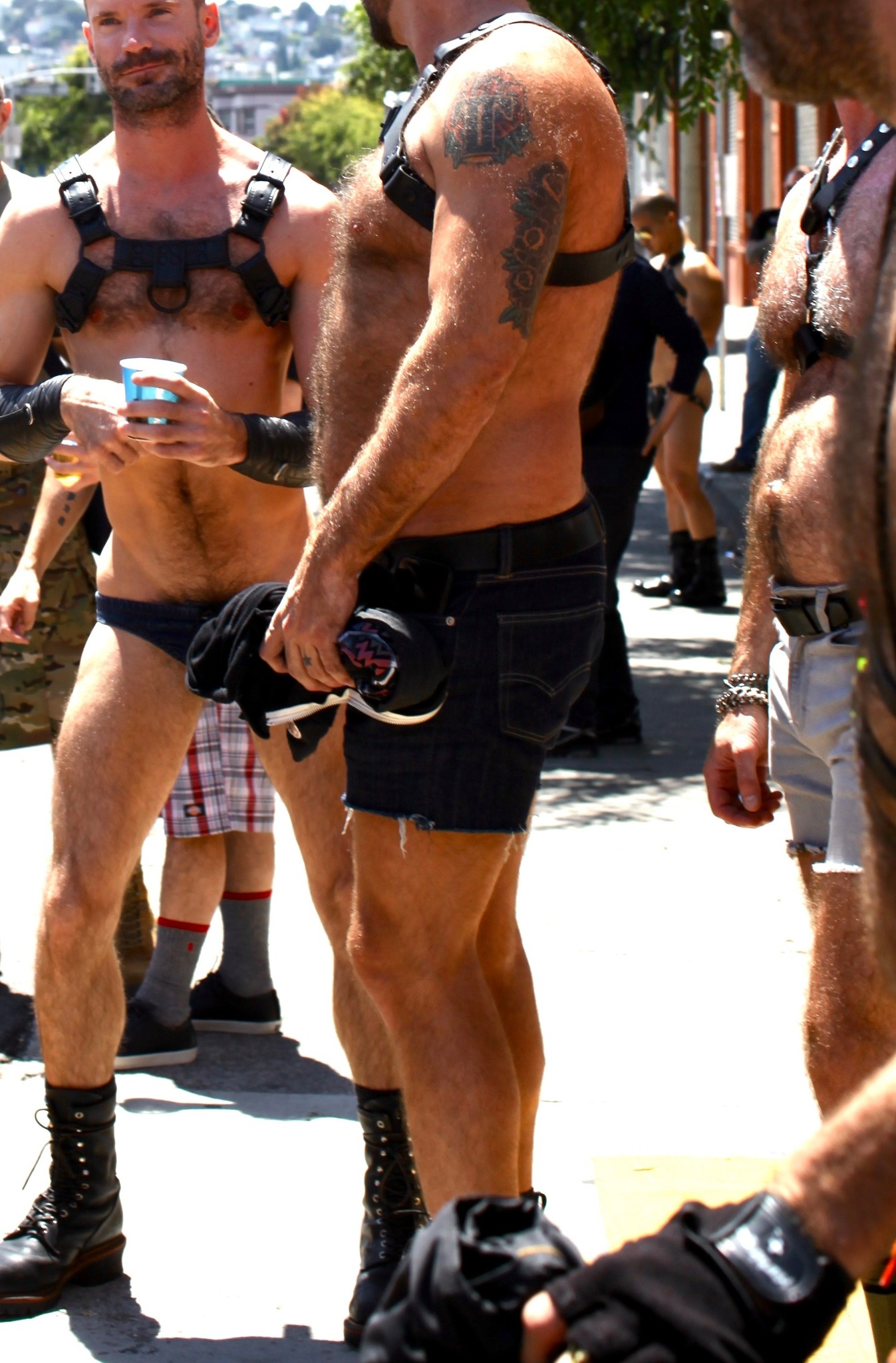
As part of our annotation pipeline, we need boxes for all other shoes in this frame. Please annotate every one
[710,457,752,473]
[0,982,27,1052]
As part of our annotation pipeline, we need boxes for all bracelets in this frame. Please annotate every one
[714,674,768,711]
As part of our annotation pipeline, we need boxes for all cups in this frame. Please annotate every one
[119,358,186,443]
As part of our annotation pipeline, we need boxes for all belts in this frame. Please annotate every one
[769,591,865,637]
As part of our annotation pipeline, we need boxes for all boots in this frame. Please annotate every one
[670,547,726,608]
[344,1106,431,1342]
[0,1095,126,1320]
[634,544,695,596]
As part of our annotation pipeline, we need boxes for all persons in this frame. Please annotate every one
[1,0,896,1362]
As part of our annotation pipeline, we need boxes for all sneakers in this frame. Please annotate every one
[190,969,281,1034]
[114,1000,199,1070]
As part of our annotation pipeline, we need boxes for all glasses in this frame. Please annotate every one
[787,186,792,191]
[634,229,654,242]
